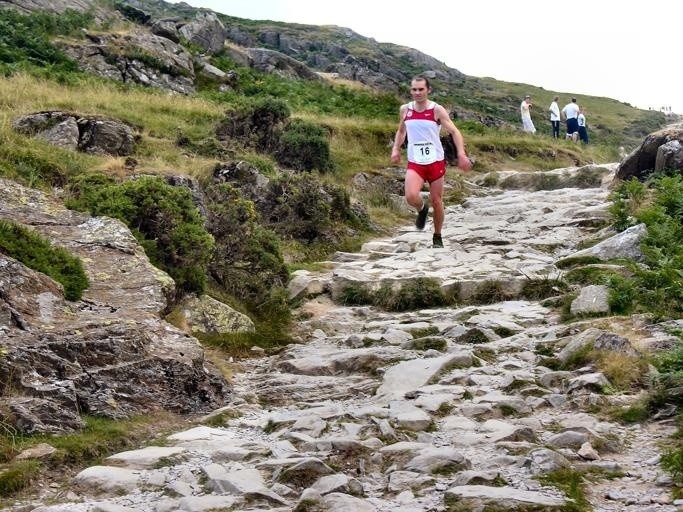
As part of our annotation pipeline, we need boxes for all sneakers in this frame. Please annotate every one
[433,233,444,248]
[416,203,428,229]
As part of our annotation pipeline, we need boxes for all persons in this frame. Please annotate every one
[577,108,590,144]
[391,75,471,249]
[550,96,561,138]
[521,95,536,134]
[560,97,579,143]
